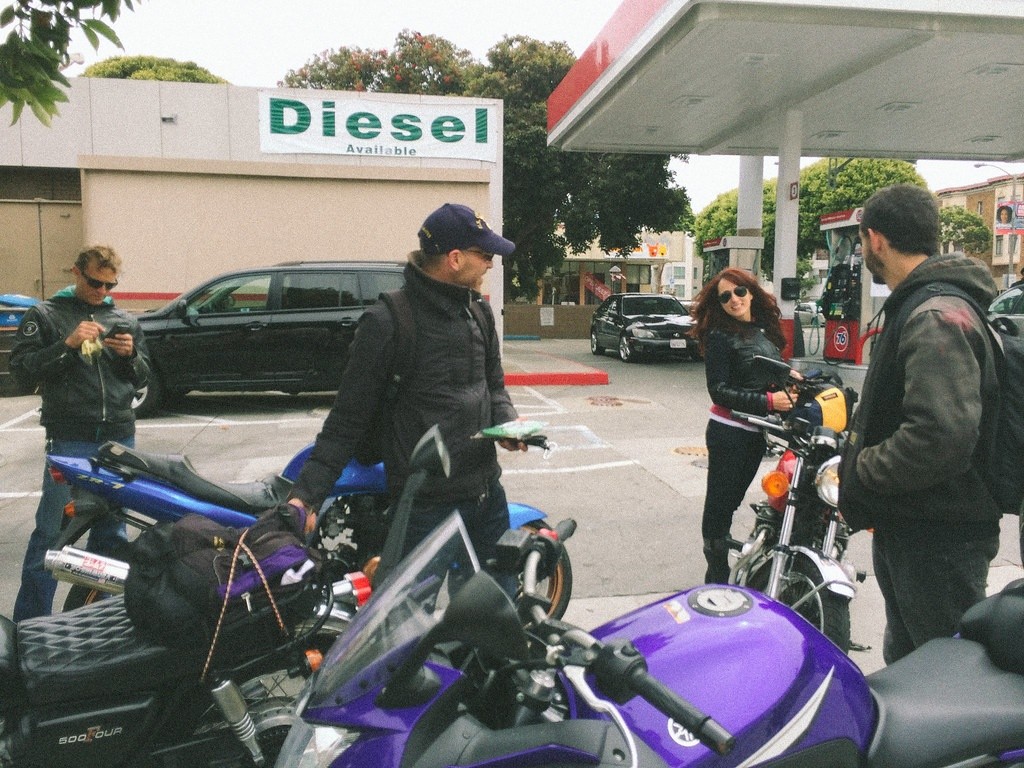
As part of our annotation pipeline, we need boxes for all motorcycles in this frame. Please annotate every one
[1,504,369,768]
[43,412,578,625]
[721,354,868,655]
[268,424,1024,768]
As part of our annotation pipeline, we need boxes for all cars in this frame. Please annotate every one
[0,292,58,397]
[590,291,702,365]
[793,300,827,329]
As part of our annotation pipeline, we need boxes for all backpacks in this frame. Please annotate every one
[125,503,327,669]
[893,281,1024,516]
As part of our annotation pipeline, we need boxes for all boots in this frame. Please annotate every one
[702,533,733,585]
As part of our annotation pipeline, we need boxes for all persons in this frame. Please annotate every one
[997,207,1012,224]
[839,186,1002,667]
[290,203,516,598]
[8,246,152,624]
[694,269,797,586]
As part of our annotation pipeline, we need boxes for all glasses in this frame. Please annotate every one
[718,286,748,304]
[79,268,119,290]
[465,249,495,261]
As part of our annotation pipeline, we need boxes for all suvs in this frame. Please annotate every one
[133,254,406,418]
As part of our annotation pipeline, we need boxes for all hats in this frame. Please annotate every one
[418,203,515,256]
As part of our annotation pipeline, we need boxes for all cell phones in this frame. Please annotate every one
[103,324,130,341]
